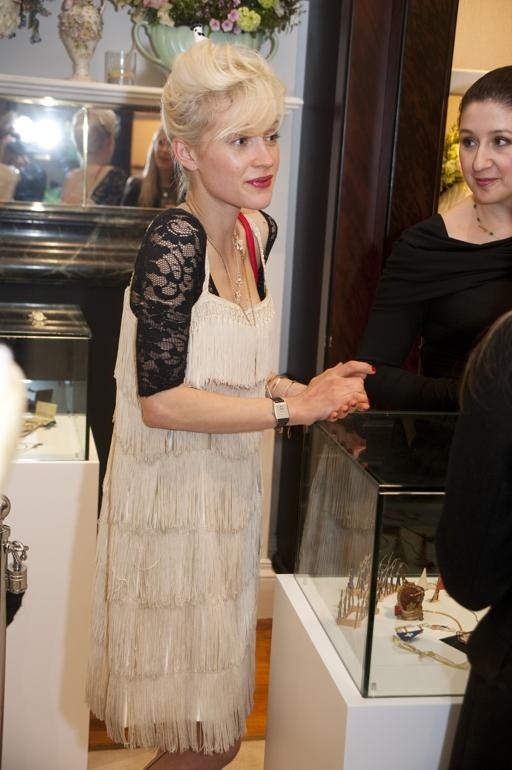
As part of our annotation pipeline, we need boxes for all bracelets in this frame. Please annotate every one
[266,375,298,398]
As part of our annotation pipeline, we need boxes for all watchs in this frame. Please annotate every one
[269,396,290,428]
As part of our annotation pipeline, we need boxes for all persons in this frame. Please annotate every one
[118,124,189,209]
[0,129,24,169]
[431,296,512,767]
[59,104,137,206]
[76,38,381,770]
[0,141,48,203]
[350,65,512,577]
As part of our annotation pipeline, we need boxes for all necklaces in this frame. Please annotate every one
[186,205,258,335]
[472,202,502,242]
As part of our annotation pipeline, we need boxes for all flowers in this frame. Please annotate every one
[120,0,302,34]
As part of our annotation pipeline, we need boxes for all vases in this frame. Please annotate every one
[59,2,106,81]
[133,20,279,77]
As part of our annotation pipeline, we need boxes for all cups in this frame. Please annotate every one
[103,50,139,86]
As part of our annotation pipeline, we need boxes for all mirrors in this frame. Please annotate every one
[0,96,191,216]
